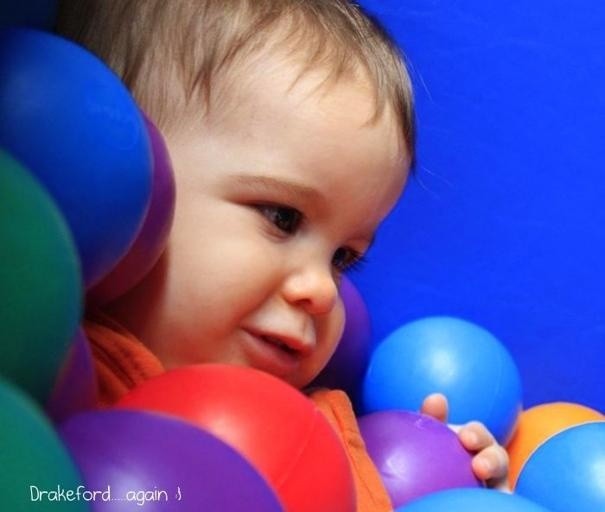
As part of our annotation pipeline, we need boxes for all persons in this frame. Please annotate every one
[0,27,605,512]
[49,1,512,512]
